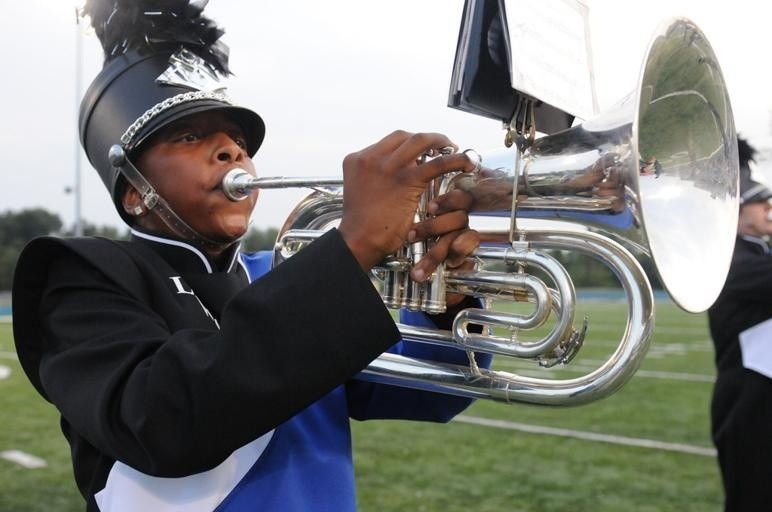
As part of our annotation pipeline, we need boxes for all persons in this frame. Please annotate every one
[11,1,487,510]
[705,134,771,512]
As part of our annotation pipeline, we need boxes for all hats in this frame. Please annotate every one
[74,44,265,227]
[740,168,772,204]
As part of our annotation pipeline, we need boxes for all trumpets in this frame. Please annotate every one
[221,14,740,407]
